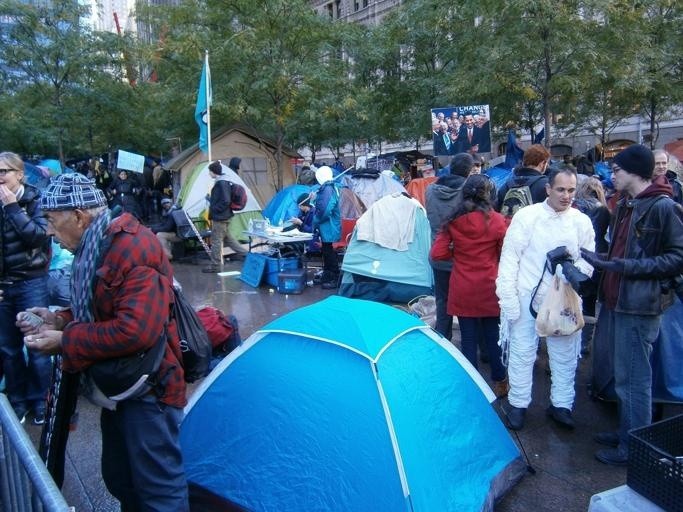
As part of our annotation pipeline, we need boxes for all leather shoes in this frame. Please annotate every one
[543,404,581,432]
[594,448,632,468]
[590,430,622,448]
[504,407,529,430]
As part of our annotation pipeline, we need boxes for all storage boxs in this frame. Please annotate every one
[236,253,297,288]
[626,412,682,512]
[276,268,307,294]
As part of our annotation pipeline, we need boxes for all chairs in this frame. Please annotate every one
[171,209,211,265]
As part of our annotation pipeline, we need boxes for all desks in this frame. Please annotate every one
[242,230,314,270]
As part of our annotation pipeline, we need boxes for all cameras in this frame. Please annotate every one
[545,245,595,298]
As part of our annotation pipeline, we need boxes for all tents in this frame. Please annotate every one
[335,192,433,302]
[171,295,537,512]
[168,160,267,255]
[263,183,365,255]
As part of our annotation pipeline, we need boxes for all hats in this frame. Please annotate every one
[208,160,224,176]
[296,192,311,207]
[613,143,655,177]
[470,152,484,170]
[38,171,109,213]
[159,195,173,206]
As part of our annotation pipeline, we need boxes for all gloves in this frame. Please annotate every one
[590,255,628,275]
[578,247,607,265]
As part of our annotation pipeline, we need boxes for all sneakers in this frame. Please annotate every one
[200,267,222,275]
[323,279,338,289]
[13,400,29,425]
[313,272,327,284]
[32,402,48,426]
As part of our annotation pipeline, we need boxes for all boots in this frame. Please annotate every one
[494,378,509,398]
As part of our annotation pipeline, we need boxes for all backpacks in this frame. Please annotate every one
[230,183,247,211]
[501,174,545,218]
[172,279,212,383]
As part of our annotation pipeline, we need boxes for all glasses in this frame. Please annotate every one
[0,167,20,176]
[608,165,622,176]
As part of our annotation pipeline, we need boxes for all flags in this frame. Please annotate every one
[195,48,213,156]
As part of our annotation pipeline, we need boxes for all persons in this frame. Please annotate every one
[479,114,490,132]
[437,122,458,155]
[471,154,485,175]
[147,197,183,263]
[1,152,52,425]
[16,172,189,512]
[455,111,490,153]
[458,114,465,124]
[556,153,577,174]
[579,146,683,467]
[110,171,139,217]
[67,156,112,189]
[140,160,165,216]
[652,149,683,204]
[433,174,512,399]
[583,147,615,191]
[432,118,440,156]
[437,112,444,123]
[199,163,255,274]
[427,153,470,342]
[451,112,459,120]
[444,117,454,132]
[281,193,321,273]
[474,115,480,128]
[311,167,343,289]
[496,145,547,215]
[453,119,461,132]
[228,157,242,174]
[495,163,596,430]
[572,174,610,355]
